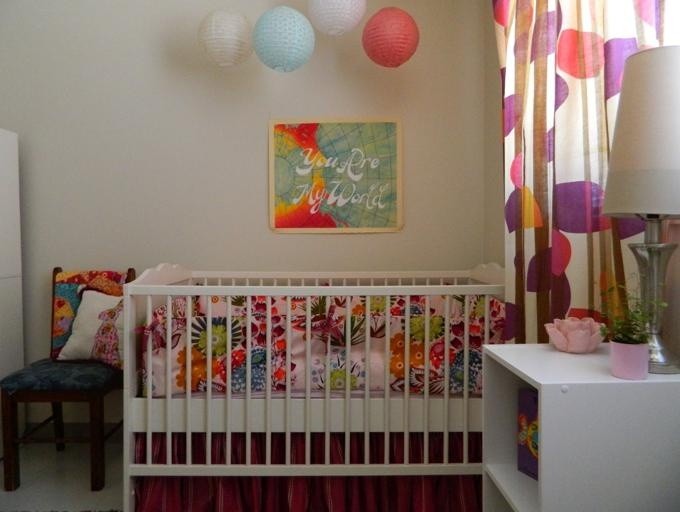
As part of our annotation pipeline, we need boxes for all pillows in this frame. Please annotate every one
[56,288,123,369]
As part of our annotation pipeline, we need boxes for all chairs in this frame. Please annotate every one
[1,266,138,493]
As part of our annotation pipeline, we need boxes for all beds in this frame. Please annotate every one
[120,261,506,512]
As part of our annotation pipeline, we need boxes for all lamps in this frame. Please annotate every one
[600,45,680,375]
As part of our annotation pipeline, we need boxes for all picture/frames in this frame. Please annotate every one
[267,116,404,234]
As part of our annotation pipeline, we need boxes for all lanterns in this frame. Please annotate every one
[308,0,367,38]
[252,5,316,74]
[198,7,253,70]
[361,9,422,71]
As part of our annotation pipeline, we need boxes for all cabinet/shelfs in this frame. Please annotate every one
[481,343,680,512]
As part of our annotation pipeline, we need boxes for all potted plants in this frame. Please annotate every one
[591,270,669,381]
